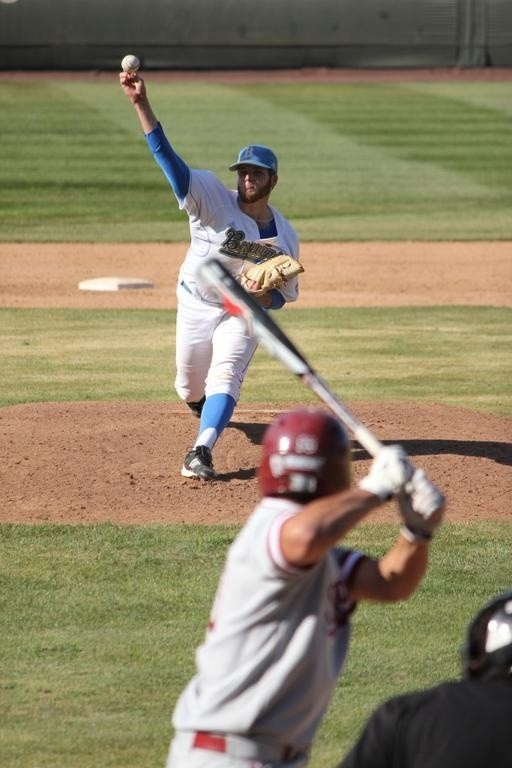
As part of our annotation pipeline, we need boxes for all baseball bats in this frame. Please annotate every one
[197,260,444,522]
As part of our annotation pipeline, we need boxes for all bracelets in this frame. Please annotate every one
[403,522,434,540]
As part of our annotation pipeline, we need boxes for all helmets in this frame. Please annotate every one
[257,405,352,500]
[457,591,512,676]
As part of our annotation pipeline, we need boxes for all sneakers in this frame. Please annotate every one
[180,444,217,481]
[186,396,207,418]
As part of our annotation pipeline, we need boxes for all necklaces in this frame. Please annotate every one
[239,201,274,224]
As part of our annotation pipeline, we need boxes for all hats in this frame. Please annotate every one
[227,144,278,172]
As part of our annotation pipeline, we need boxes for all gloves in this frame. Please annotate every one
[357,443,414,502]
[396,466,445,544]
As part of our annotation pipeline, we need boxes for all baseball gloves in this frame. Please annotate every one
[240,253,304,297]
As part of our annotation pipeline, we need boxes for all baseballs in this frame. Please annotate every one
[121,55,140,72]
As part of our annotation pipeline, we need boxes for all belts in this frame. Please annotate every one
[179,279,191,294]
[194,730,307,767]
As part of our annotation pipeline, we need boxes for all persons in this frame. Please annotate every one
[334,590,512,766]
[119,70,302,480]
[163,409,446,767]
